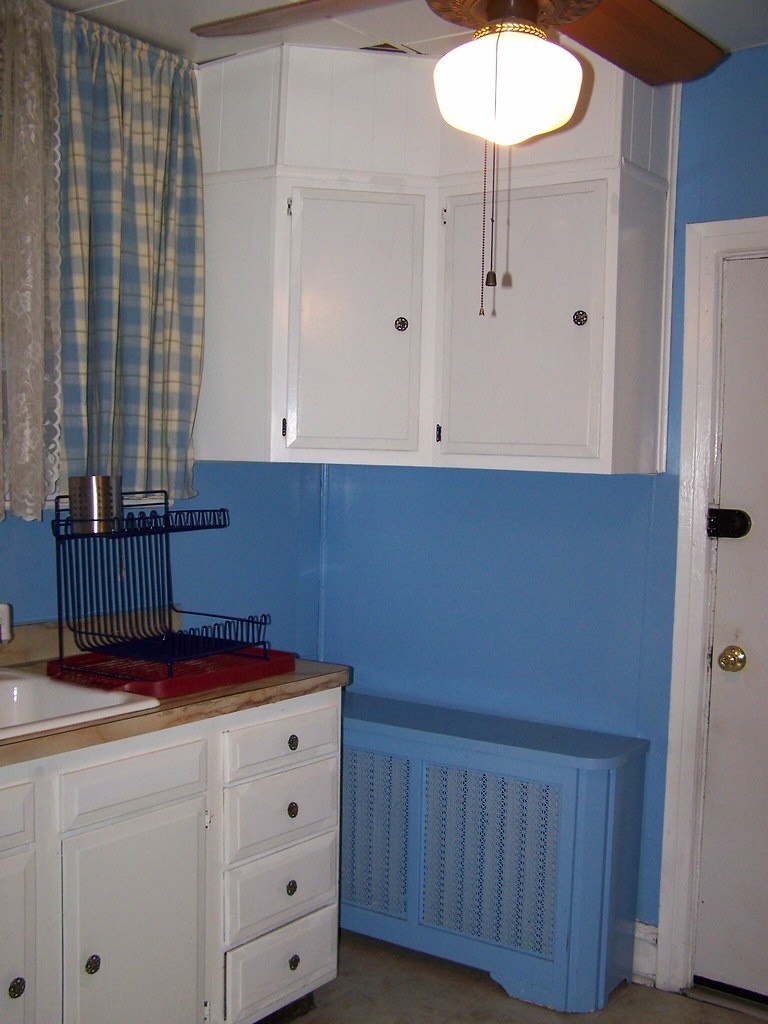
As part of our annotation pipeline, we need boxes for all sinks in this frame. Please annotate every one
[0,671,159,745]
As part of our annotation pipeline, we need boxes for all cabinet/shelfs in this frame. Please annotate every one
[193,41,439,470]
[433,30,684,475]
[0,686,341,1024]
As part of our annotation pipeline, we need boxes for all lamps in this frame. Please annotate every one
[432,0,583,147]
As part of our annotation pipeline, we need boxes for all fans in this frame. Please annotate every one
[190,0,729,87]
